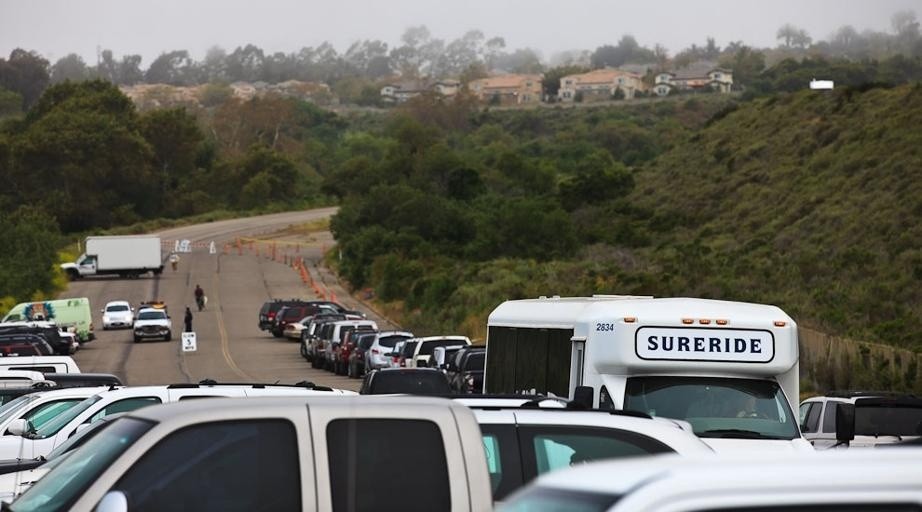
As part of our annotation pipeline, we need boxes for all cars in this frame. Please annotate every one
[99,300,136,331]
[136,300,168,314]
[494,449,922,512]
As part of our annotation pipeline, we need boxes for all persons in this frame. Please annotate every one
[193,284,208,313]
[183,306,195,334]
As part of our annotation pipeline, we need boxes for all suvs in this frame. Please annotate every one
[132,307,172,343]
[0,294,921,511]
[799,389,922,447]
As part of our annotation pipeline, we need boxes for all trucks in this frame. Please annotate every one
[481,294,818,455]
[60,234,164,281]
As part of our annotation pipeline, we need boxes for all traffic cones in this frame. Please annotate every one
[225,237,339,303]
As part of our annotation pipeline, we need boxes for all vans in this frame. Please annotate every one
[0,296,97,347]
